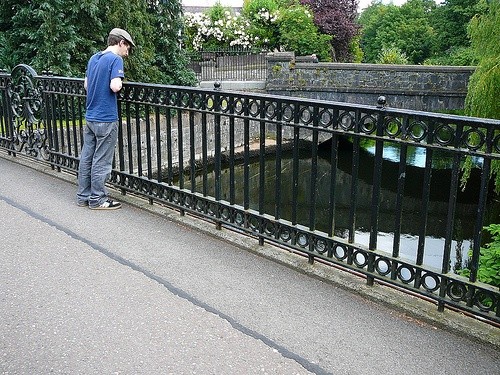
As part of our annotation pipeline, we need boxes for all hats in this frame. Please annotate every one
[109,28,136,47]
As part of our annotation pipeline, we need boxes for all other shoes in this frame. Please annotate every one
[76,197,87,206]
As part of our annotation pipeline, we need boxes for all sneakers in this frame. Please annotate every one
[89,199,122,210]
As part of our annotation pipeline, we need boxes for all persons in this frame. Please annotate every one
[76,28,136,210]
